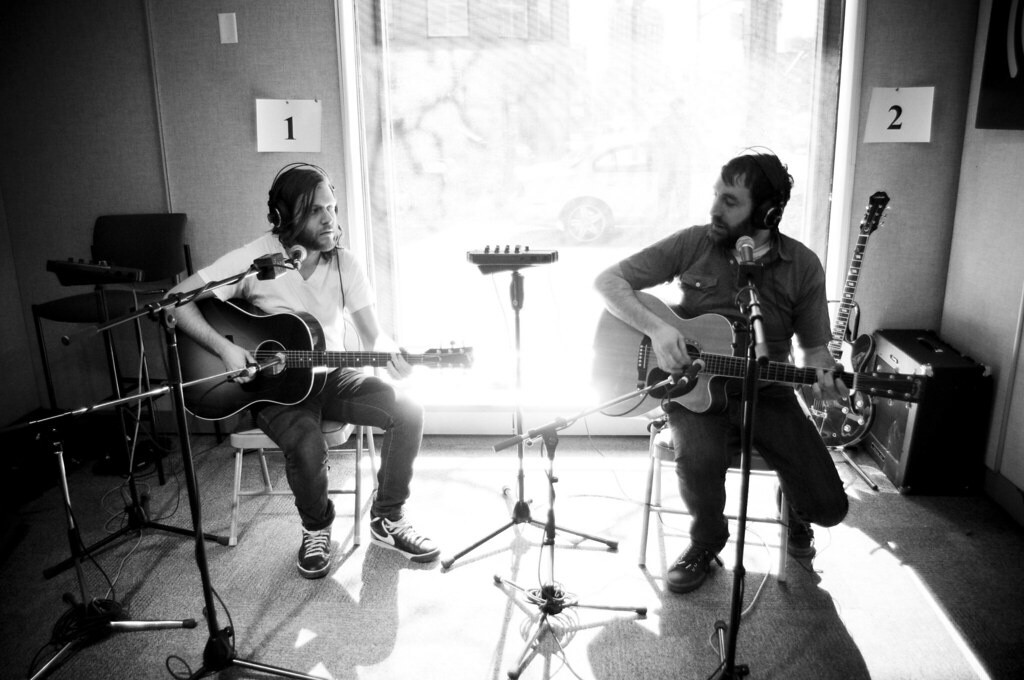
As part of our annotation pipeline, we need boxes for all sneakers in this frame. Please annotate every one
[370,512,440,564]
[296,524,331,580]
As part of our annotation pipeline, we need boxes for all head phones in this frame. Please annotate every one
[739,146,784,230]
[265,163,338,230]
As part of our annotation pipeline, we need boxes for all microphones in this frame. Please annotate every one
[678,359,705,387]
[290,244,308,270]
[239,351,287,378]
[735,235,765,289]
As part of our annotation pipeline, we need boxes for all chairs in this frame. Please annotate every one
[227,315,380,547]
[639,414,789,584]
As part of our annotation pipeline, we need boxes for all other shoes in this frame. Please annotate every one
[777,485,814,556]
[666,543,724,591]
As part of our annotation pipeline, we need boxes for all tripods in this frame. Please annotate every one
[0,255,326,680]
[442,267,684,680]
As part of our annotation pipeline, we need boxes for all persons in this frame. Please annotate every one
[160,161,441,579]
[593,148,851,595]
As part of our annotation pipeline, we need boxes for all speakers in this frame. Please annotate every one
[861,328,995,501]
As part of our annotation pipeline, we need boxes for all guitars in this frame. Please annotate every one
[591,292,932,415]
[168,289,472,421]
[796,190,890,449]
